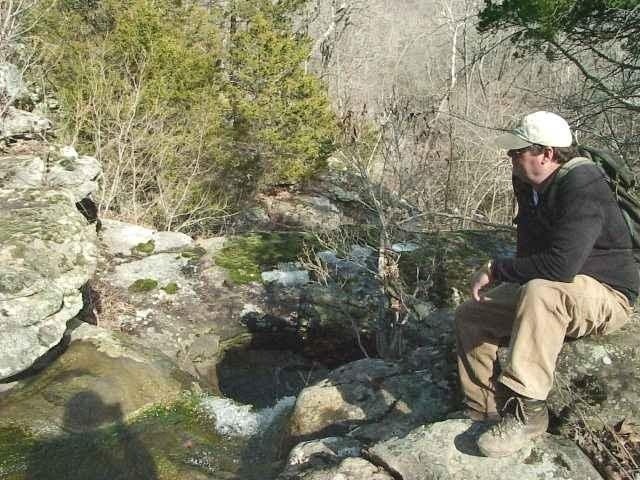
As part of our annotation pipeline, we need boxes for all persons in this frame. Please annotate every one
[445,109,639,460]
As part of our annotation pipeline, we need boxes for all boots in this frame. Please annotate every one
[475,397,551,459]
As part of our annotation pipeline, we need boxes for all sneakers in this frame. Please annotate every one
[440,402,502,425]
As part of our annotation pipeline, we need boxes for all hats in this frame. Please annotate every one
[498,110,573,152]
